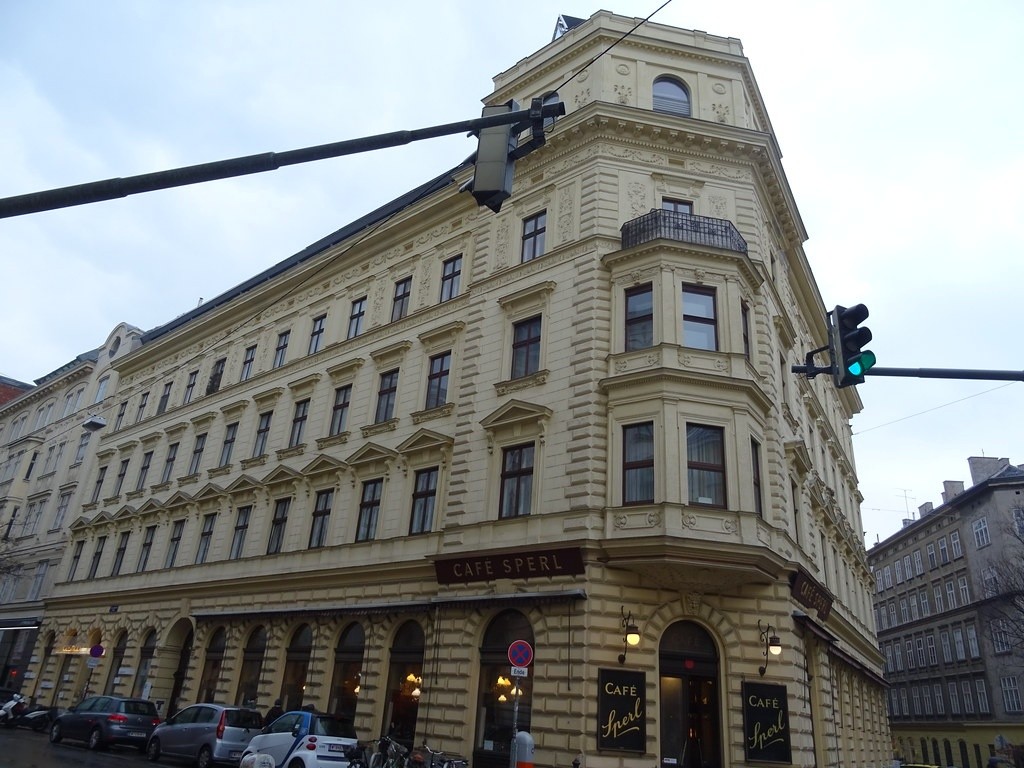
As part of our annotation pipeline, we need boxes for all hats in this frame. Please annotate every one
[274,699,281,705]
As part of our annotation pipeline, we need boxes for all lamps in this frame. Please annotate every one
[757,619,783,677]
[495,662,525,707]
[404,668,423,702]
[618,605,642,663]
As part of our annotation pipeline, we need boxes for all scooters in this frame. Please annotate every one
[0,693,52,734]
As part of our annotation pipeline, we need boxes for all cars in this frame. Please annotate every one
[146,703,263,768]
[240,710,360,768]
[48,696,161,752]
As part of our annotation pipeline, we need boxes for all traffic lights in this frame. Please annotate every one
[826,301,876,386]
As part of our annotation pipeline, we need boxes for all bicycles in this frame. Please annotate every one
[346,734,468,768]
